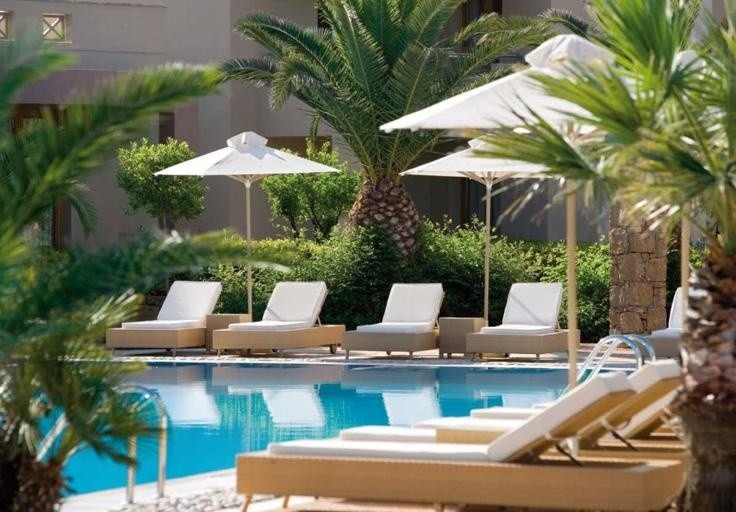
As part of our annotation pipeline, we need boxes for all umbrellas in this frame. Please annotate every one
[637,47,724,332]
[152,129,342,319]
[400,136,550,323]
[378,32,649,390]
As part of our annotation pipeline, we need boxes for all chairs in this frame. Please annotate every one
[212,281,346,355]
[236,358,686,512]
[105,282,222,357]
[341,281,445,358]
[466,281,582,360]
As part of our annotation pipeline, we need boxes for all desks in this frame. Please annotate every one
[439,317,483,358]
[206,313,251,354]
